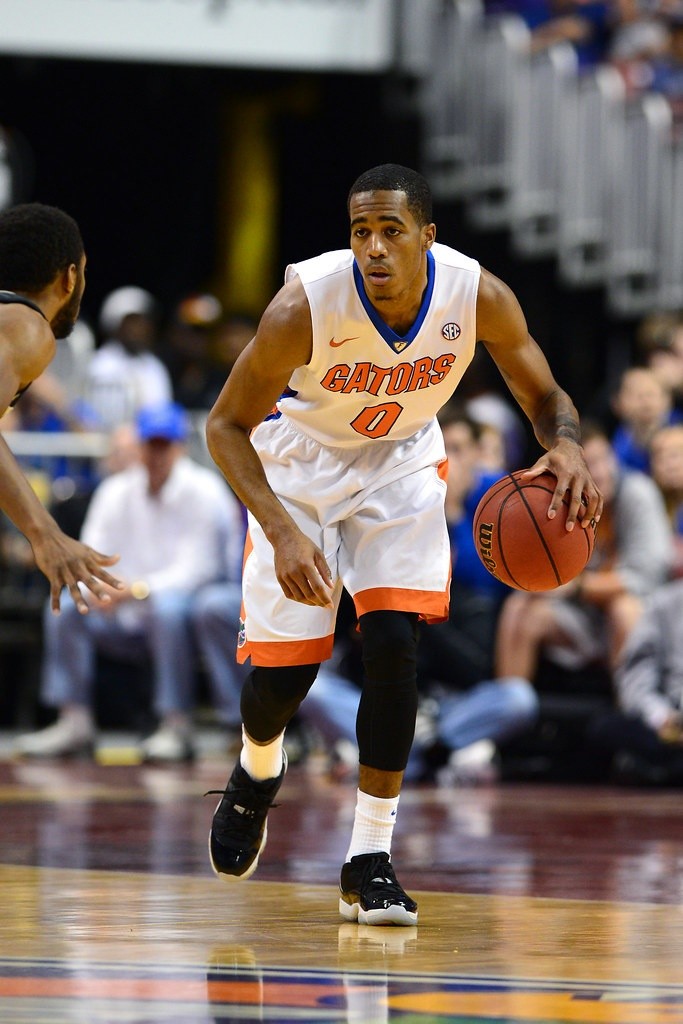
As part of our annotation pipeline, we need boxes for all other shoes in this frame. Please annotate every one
[17,718,96,757]
[281,734,304,764]
[145,720,194,761]
[436,741,496,786]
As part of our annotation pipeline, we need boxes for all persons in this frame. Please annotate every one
[463,0,683,121]
[205,164,604,926]
[190,307,683,783]
[0,204,124,618]
[89,287,172,427]
[18,400,244,759]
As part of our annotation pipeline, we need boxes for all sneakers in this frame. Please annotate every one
[339,851,418,926]
[203,747,288,880]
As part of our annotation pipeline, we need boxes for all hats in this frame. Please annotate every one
[101,286,149,329]
[136,401,188,443]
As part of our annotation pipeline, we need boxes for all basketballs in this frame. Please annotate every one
[470,468,595,594]
